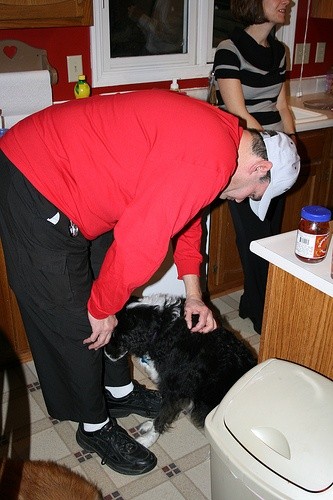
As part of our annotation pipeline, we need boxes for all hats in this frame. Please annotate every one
[248,130,300,222]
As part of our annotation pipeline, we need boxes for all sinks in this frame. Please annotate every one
[288,103,328,125]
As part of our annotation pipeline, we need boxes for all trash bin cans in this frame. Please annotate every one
[204,357,333,500]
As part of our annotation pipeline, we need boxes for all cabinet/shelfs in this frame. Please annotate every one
[249,264,333,382]
[1,127,333,365]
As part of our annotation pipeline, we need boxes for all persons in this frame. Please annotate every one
[0,89,301,477]
[209,0,296,336]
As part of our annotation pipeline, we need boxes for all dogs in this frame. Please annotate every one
[103,294,258,450]
[0,457,104,500]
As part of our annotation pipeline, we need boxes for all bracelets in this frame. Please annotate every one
[287,133,296,137]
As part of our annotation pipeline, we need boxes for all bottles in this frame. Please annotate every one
[171,78,179,92]
[75,75,90,98]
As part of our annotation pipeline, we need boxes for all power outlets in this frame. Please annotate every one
[294,43,310,65]
[66,54,83,83]
[315,42,325,63]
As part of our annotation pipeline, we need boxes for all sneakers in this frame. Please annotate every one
[76,416,157,476]
[103,377,193,421]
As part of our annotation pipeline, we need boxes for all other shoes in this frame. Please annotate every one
[238,309,260,336]
